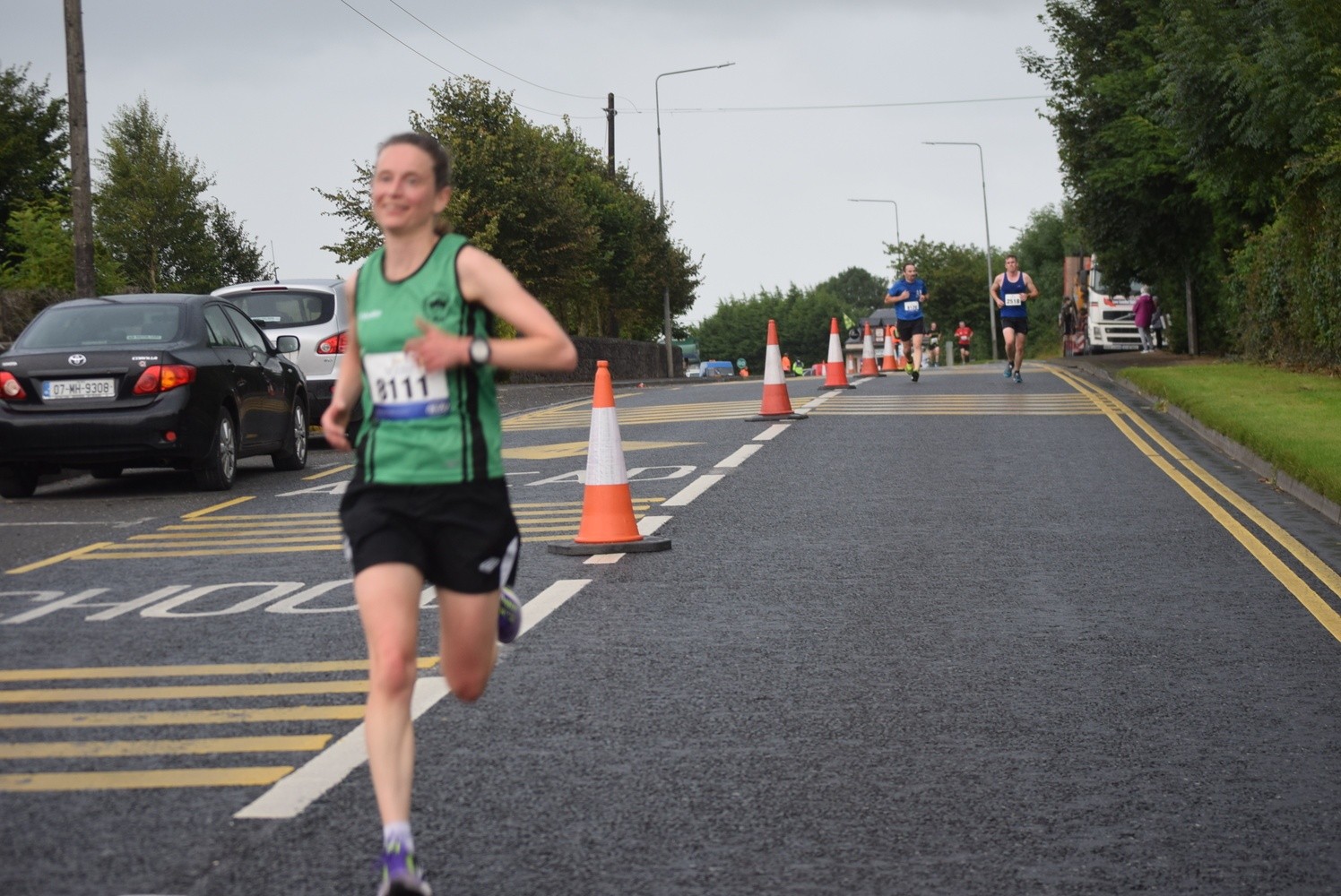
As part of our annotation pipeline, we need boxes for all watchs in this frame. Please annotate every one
[468,334,492,368]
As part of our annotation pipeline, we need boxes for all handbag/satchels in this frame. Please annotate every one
[1160,314,1172,329]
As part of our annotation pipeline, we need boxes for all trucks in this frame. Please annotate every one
[1078,253,1173,352]
[681,354,736,379]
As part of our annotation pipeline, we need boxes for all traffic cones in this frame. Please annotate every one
[820,359,827,378]
[815,317,855,390]
[877,323,908,373]
[850,321,889,377]
[550,360,675,554]
[847,354,857,376]
[743,319,811,420]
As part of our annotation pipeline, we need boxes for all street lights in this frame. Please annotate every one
[922,141,998,360]
[848,198,903,277]
[653,62,745,382]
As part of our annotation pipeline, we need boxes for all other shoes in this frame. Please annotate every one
[935,363,939,367]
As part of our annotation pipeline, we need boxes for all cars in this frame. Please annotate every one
[1,291,311,493]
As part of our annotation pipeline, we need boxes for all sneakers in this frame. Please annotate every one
[906,362,914,376]
[1141,350,1149,353]
[1012,371,1023,384]
[1149,349,1155,353]
[1004,365,1013,379]
[497,588,522,643]
[378,839,431,896]
[911,369,920,383]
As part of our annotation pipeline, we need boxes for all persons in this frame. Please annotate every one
[793,356,805,376]
[739,367,749,378]
[321,133,579,896]
[884,263,929,382]
[782,352,791,377]
[991,255,1038,383]
[954,321,973,364]
[925,323,941,365]
[1133,284,1163,354]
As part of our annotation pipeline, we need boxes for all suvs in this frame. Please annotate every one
[208,277,362,439]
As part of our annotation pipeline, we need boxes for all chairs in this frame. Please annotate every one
[140,320,177,337]
[249,296,293,323]
